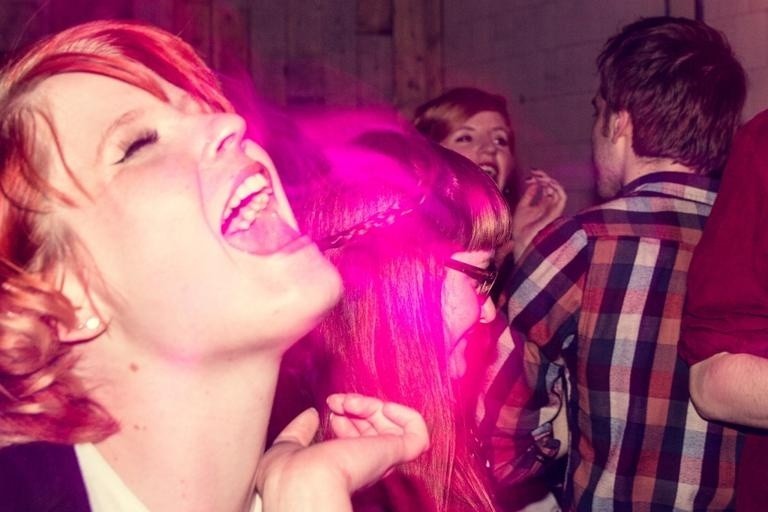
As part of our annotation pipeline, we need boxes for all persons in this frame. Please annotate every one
[677,106,768,512]
[485,16,748,512]
[0,19,433,512]
[412,85,570,316]
[257,130,514,512]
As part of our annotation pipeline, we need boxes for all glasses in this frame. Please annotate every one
[446,258,498,296]
